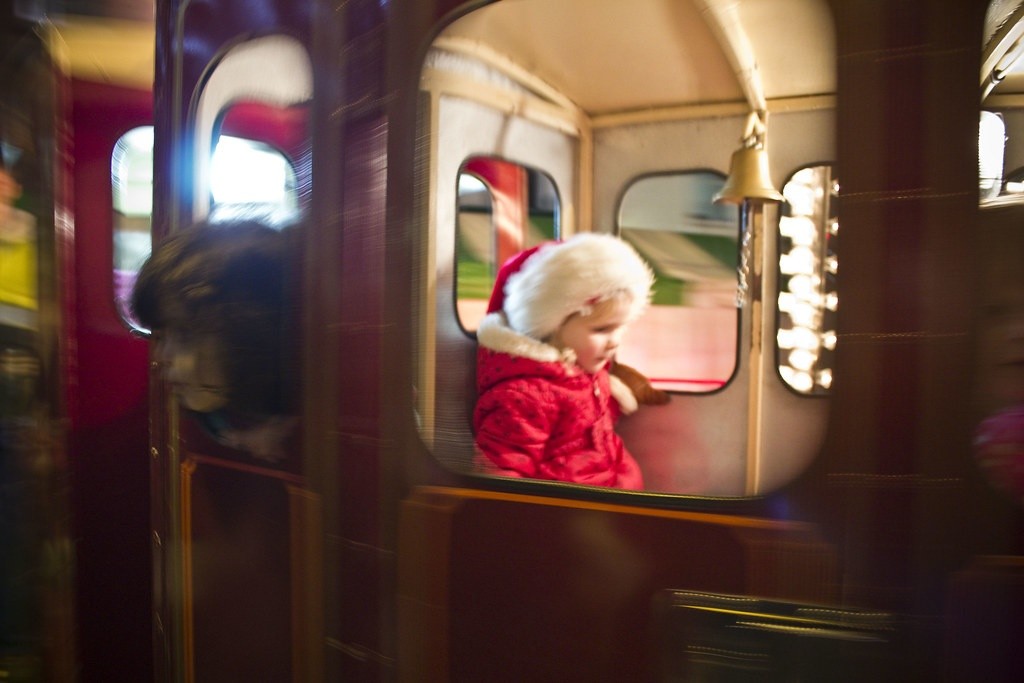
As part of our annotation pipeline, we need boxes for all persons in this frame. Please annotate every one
[473,231,672,491]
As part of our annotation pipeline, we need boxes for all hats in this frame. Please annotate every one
[485,231,654,338]
